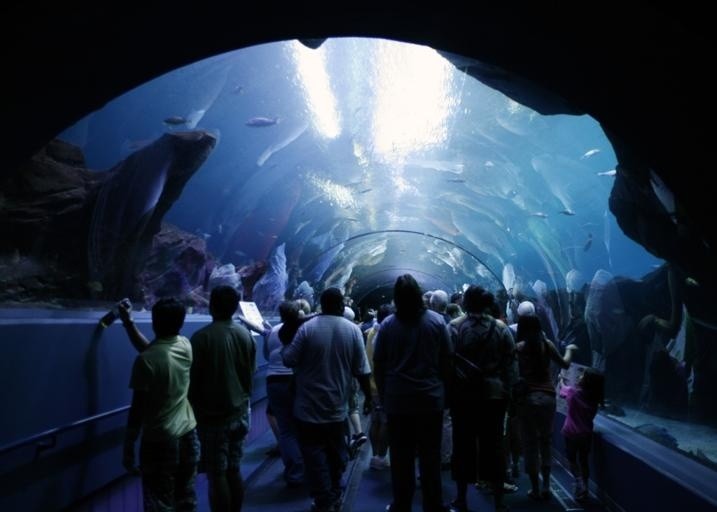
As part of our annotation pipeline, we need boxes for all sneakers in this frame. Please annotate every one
[348,433,367,460]
[369,455,390,470]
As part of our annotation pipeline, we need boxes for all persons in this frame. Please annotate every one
[115,275,611,512]
[541,260,717,420]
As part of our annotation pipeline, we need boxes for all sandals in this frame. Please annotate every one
[504,485,518,492]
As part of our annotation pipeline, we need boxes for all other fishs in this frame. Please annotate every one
[583,239,593,251]
[580,148,600,160]
[358,189,373,193]
[559,209,576,216]
[596,170,617,177]
[528,212,549,219]
[229,84,243,95]
[164,115,192,124]
[446,179,466,183]
[346,216,359,221]
[244,116,279,126]
[310,217,342,237]
